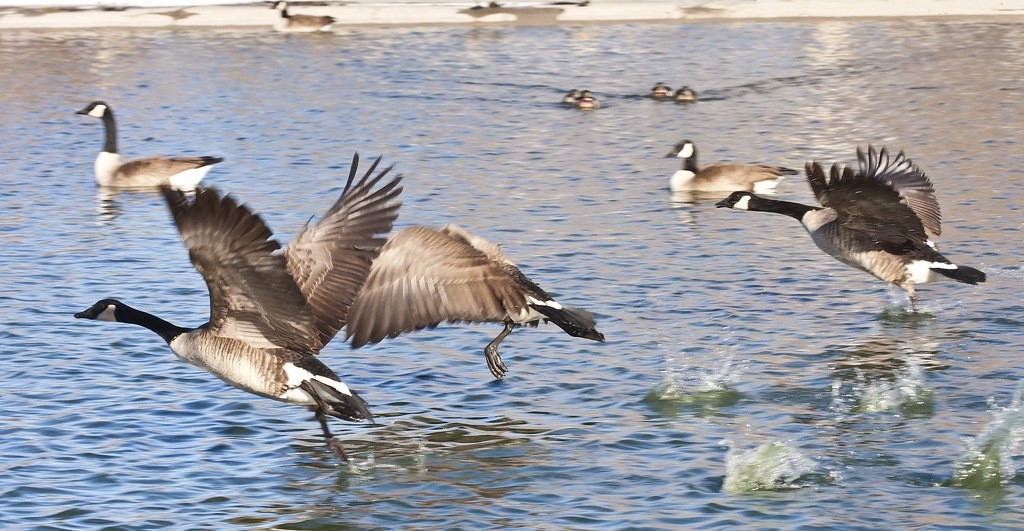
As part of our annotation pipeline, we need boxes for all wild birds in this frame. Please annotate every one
[74,153,405,460]
[663,139,800,193]
[649,81,673,97]
[75,101,225,192]
[269,1,336,33]
[675,85,697,100]
[563,89,600,109]
[714,144,987,309]
[342,224,606,380]
